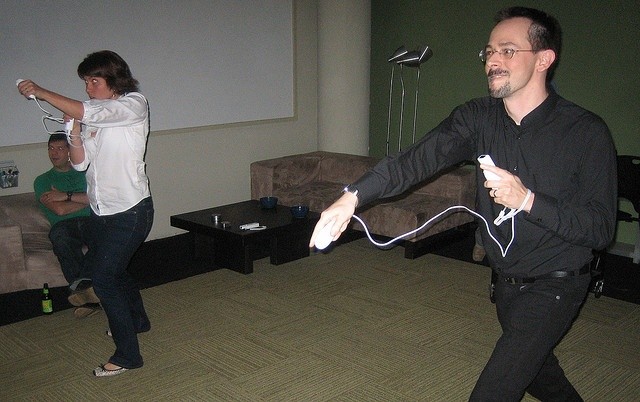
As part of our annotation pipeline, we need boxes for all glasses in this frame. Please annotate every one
[479,47,536,61]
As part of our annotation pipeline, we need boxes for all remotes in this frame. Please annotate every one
[239,222,260,230]
[249,225,268,231]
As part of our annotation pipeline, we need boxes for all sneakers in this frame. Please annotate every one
[74,300,99,319]
[93,363,131,377]
[105,331,112,337]
[68,282,102,306]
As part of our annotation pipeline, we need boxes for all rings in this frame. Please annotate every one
[493,190,497,199]
[47,195,50,200]
[25,88,29,93]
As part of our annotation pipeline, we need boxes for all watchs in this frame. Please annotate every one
[66,191,72,201]
[340,183,361,207]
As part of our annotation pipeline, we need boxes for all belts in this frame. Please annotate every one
[506,267,590,285]
[142,197,153,203]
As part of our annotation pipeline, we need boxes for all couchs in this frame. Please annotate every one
[248,151,478,259]
[0,192,70,295]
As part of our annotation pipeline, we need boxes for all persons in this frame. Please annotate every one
[33,129,106,320]
[307,8,619,400]
[17,50,154,378]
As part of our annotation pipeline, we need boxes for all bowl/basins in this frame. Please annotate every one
[260,196,278,209]
[290,205,309,219]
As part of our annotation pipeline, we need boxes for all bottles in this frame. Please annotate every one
[40,283,55,315]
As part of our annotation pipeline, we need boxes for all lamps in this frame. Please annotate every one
[413,44,434,143]
[396,50,420,152]
[387,44,409,157]
